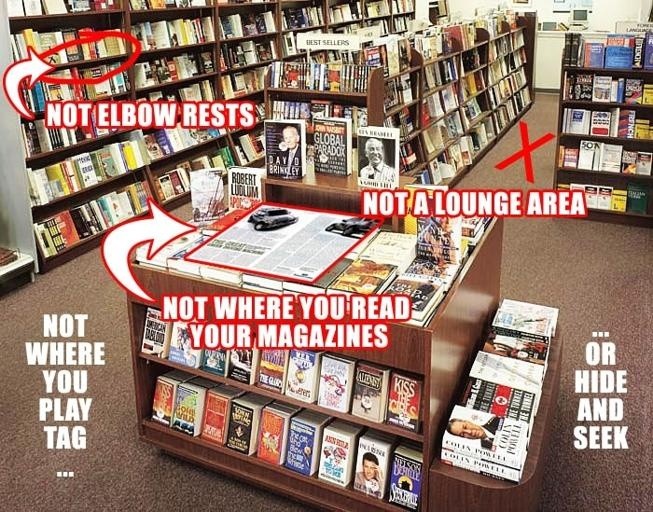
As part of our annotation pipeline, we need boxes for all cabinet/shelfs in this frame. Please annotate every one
[0,1,653,511]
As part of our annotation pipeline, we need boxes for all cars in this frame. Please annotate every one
[324,218,378,236]
[248,207,298,230]
